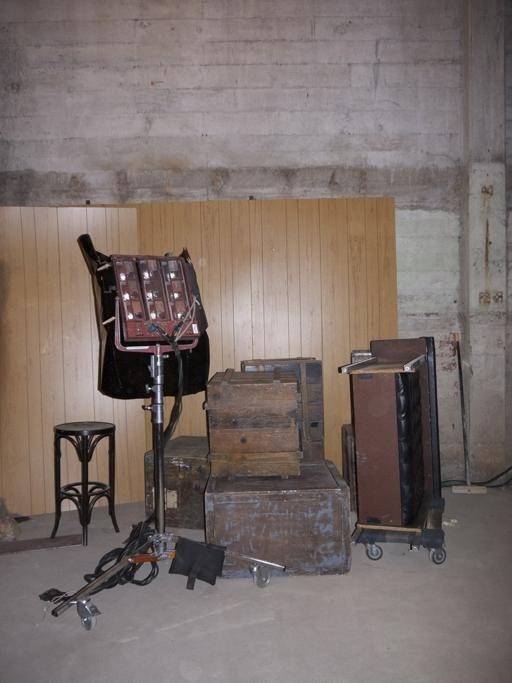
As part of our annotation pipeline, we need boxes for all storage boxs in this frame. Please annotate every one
[205,458,353,581]
[203,370,303,480]
[241,358,324,456]
[144,436,211,529]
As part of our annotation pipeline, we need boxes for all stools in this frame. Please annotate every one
[51,422,120,548]
